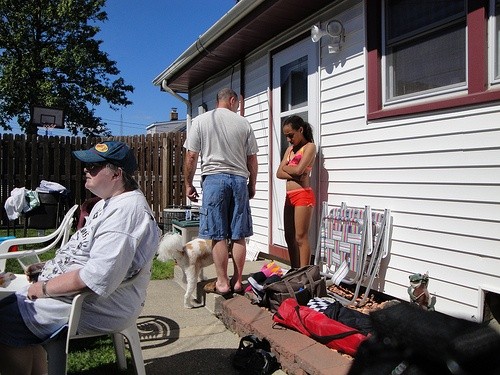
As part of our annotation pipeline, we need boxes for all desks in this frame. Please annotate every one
[163,208,200,246]
[7,192,62,251]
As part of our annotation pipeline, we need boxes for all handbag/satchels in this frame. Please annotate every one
[232,335,281,375]
[272,297,369,356]
[262,265,328,313]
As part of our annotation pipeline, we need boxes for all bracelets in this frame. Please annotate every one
[42,279,51,298]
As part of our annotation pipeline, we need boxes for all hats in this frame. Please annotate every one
[72,142,133,167]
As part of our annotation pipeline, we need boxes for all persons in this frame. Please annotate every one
[276,116,316,269]
[183,88,260,295]
[0,142,158,375]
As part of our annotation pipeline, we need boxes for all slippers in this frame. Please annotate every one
[202,280,233,296]
[229,279,246,293]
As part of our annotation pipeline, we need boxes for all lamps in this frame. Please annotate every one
[310,19,346,54]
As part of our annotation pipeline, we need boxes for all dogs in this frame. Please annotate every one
[156,232,215,308]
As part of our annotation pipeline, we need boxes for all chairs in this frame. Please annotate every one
[0,205,79,291]
[45,244,160,375]
[314,200,392,307]
[77,197,102,229]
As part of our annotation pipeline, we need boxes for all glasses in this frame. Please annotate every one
[85,162,108,170]
